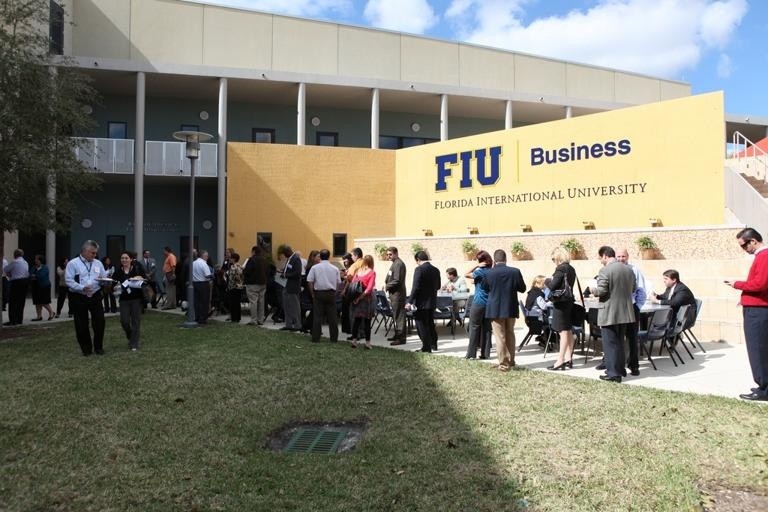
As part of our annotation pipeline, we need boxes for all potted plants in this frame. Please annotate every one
[511,244,529,261]
[372,243,388,261]
[635,237,660,261]
[563,238,585,260]
[463,241,481,261]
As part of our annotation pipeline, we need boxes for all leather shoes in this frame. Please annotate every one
[739,387,767,401]
[596,363,640,383]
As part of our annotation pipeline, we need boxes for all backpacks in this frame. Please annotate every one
[345,279,363,303]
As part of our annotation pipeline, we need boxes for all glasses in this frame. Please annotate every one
[740,240,751,249]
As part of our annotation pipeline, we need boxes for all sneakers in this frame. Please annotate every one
[2,302,177,356]
[431,344,439,350]
[389,339,406,345]
[388,336,397,341]
[223,315,373,350]
[415,347,431,353]
[462,344,516,372]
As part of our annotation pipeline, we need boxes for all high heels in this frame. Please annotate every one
[547,361,573,371]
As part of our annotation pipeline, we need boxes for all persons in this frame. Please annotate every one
[524,246,646,382]
[405,251,441,353]
[460,250,491,359]
[440,268,466,327]
[651,269,697,351]
[384,247,406,345]
[724,228,768,400]
[480,249,526,371]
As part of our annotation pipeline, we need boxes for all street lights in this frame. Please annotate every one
[171,129,218,330]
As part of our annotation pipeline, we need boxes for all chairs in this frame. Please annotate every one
[153,279,343,326]
[374,277,707,371]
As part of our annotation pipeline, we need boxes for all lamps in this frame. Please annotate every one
[646,218,662,227]
[581,220,595,231]
[464,226,479,234]
[515,222,532,233]
[422,227,431,235]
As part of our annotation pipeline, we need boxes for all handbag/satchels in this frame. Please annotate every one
[141,283,155,302]
[547,273,573,304]
[572,303,587,328]
